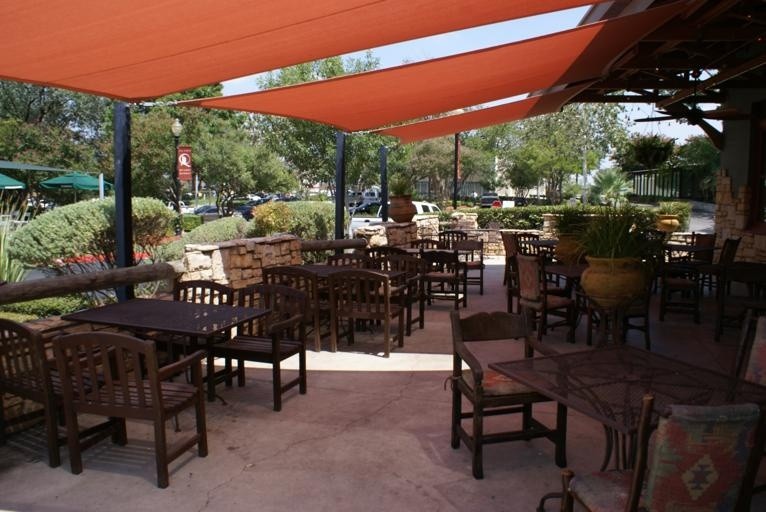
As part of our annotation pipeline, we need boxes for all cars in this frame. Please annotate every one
[450,193,610,206]
[162,192,305,223]
[26,196,52,209]
[345,190,389,214]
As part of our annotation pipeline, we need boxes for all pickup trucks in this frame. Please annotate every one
[347,199,441,241]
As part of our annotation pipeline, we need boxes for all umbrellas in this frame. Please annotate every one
[0,173,28,193]
[39,170,114,206]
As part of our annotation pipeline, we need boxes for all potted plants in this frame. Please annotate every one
[373,163,430,222]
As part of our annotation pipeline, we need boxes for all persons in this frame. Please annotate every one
[471,190,478,203]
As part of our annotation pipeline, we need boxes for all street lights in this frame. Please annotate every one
[170,116,183,236]
